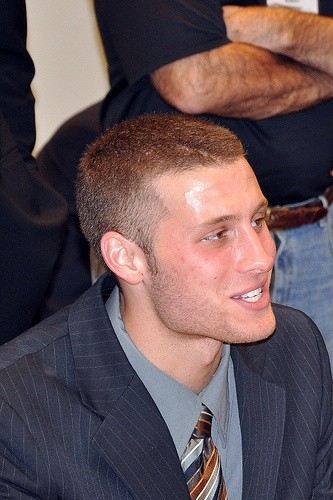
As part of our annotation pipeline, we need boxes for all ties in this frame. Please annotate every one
[180,403,228,499]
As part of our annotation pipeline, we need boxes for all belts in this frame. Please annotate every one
[264,184,332,231]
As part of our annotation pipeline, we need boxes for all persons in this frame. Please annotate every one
[0,116,332,499]
[94,0,331,366]
